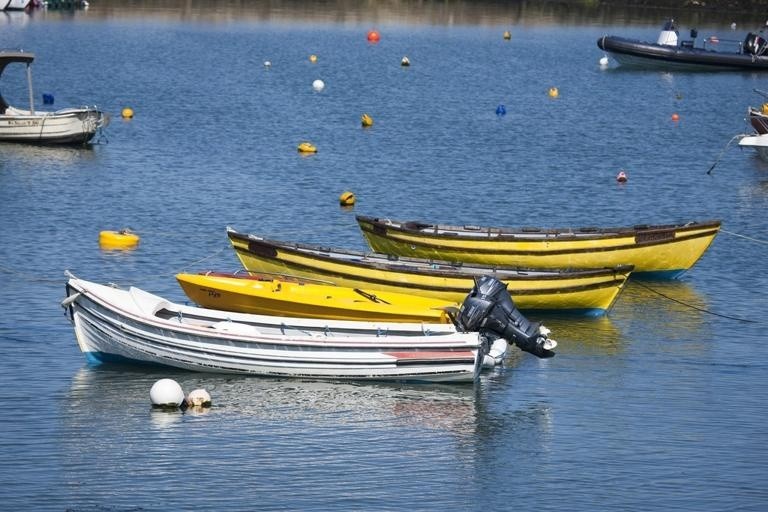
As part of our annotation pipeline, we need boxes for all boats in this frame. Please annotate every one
[175,272,467,324]
[354,212,724,285]
[0,49,110,145]
[61,274,559,382]
[737,106,768,161]
[226,224,638,317]
[597,33,768,73]
